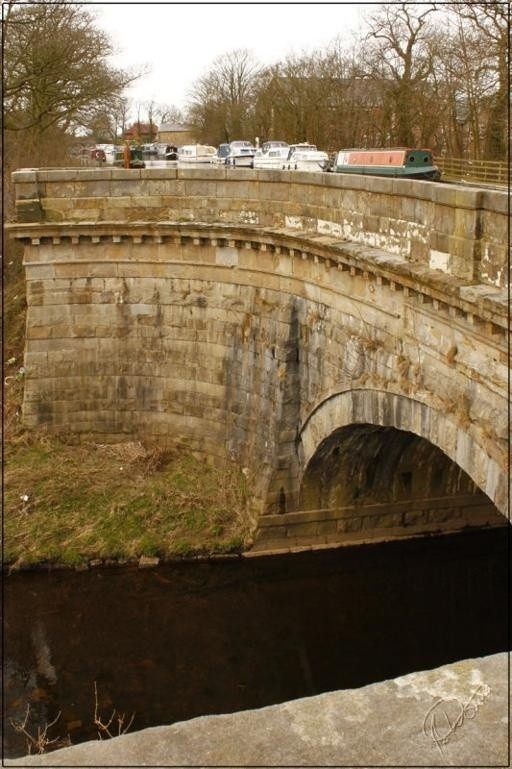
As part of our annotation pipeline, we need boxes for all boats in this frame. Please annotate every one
[157,139,442,182]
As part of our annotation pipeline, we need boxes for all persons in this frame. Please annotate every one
[122,140,131,168]
